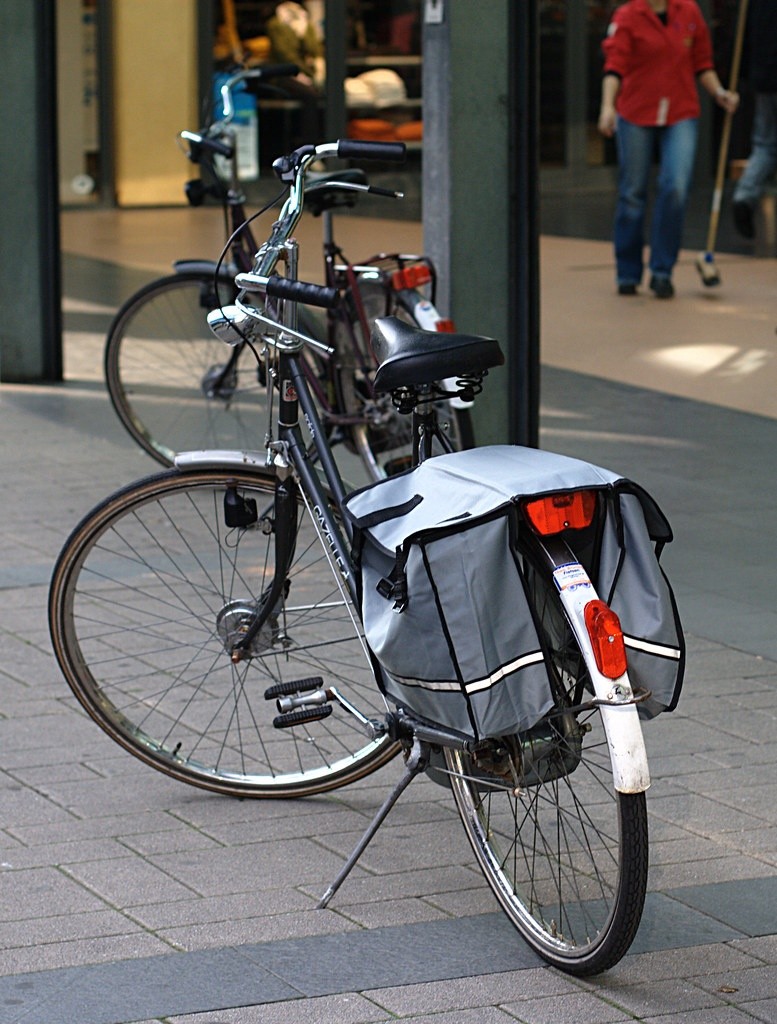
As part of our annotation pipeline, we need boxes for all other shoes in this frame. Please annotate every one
[734,202,756,237]
[620,284,634,293]
[651,276,673,297]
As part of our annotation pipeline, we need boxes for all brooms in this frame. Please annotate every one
[692,3,752,286]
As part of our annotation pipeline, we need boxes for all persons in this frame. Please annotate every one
[598,1,740,300]
[733,0,777,239]
[253,1,414,148]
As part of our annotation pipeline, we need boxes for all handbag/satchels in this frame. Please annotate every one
[341,444,686,745]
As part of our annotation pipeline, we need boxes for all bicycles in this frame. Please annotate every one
[48,137,655,978]
[103,62,474,497]
